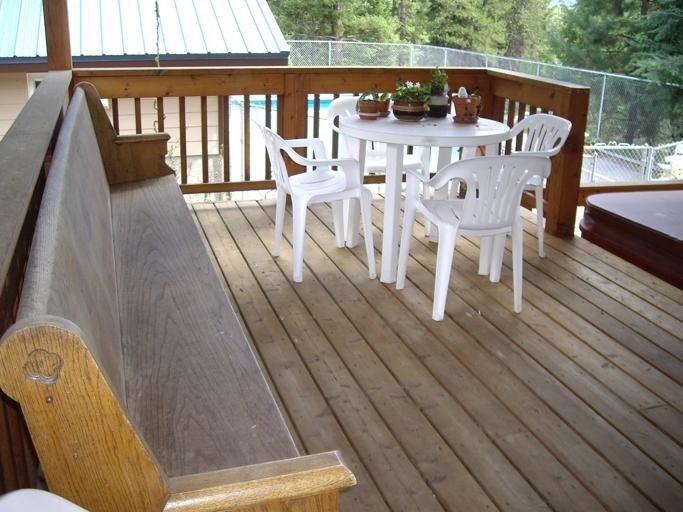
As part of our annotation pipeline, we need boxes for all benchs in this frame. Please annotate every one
[0,79,359,510]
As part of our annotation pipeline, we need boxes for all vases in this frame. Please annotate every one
[357,98,379,120]
[449,95,484,125]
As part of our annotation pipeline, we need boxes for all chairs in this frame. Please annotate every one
[256,121,377,284]
[448,110,576,281]
[327,94,437,241]
[393,149,552,325]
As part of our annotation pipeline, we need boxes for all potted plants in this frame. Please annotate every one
[388,78,433,123]
[424,63,452,120]
[354,87,391,119]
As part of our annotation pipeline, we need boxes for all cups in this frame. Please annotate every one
[360,101,377,120]
[452,94,480,118]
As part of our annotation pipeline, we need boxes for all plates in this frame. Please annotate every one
[452,116,478,124]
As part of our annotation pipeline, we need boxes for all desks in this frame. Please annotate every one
[333,113,515,288]
